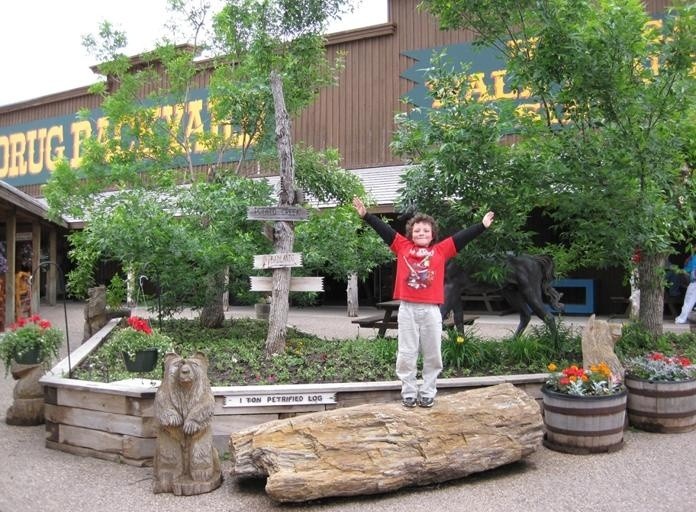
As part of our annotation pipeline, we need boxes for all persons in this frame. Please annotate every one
[352,195,495,407]
[666,263,683,314]
[627,247,642,321]
[675,245,696,324]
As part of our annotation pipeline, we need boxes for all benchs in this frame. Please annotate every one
[445,313,481,328]
[351,312,385,328]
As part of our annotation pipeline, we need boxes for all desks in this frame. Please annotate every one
[374,299,400,340]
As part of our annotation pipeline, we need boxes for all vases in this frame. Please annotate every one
[13,347,41,363]
[120,346,157,373]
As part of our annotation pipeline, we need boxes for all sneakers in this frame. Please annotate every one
[402,397,417,406]
[419,398,433,407]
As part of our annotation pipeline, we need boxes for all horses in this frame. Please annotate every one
[388,212,565,351]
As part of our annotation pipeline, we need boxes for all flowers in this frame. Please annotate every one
[0,312,62,376]
[100,313,176,363]
[624,349,696,382]
[543,360,623,397]
